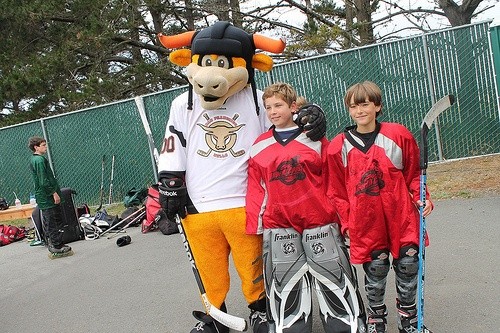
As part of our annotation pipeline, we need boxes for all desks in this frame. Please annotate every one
[0,204,38,221]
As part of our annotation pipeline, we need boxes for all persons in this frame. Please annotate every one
[324,80,436,333]
[295,96,307,112]
[244,81,367,333]
[27,136,73,258]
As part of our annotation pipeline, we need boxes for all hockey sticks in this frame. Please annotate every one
[135,95,248,332]
[100,206,144,239]
[417,93,456,333]
[96,153,115,211]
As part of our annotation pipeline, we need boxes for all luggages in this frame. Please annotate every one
[41,188,85,246]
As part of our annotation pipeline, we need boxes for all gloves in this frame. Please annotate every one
[116,236,131,247]
[297,102,326,141]
[158,178,188,222]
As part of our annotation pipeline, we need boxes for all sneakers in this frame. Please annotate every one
[248,297,269,333]
[366,304,388,333]
[190,302,230,333]
[396,298,432,333]
[48,244,73,260]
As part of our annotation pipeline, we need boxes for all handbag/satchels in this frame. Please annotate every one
[76,184,179,235]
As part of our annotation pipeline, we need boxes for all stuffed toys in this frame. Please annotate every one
[156,22,327,333]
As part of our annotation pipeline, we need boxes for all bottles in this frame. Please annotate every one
[29,192,36,207]
[15,198,21,209]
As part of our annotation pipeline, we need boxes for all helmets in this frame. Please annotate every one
[83,224,99,241]
